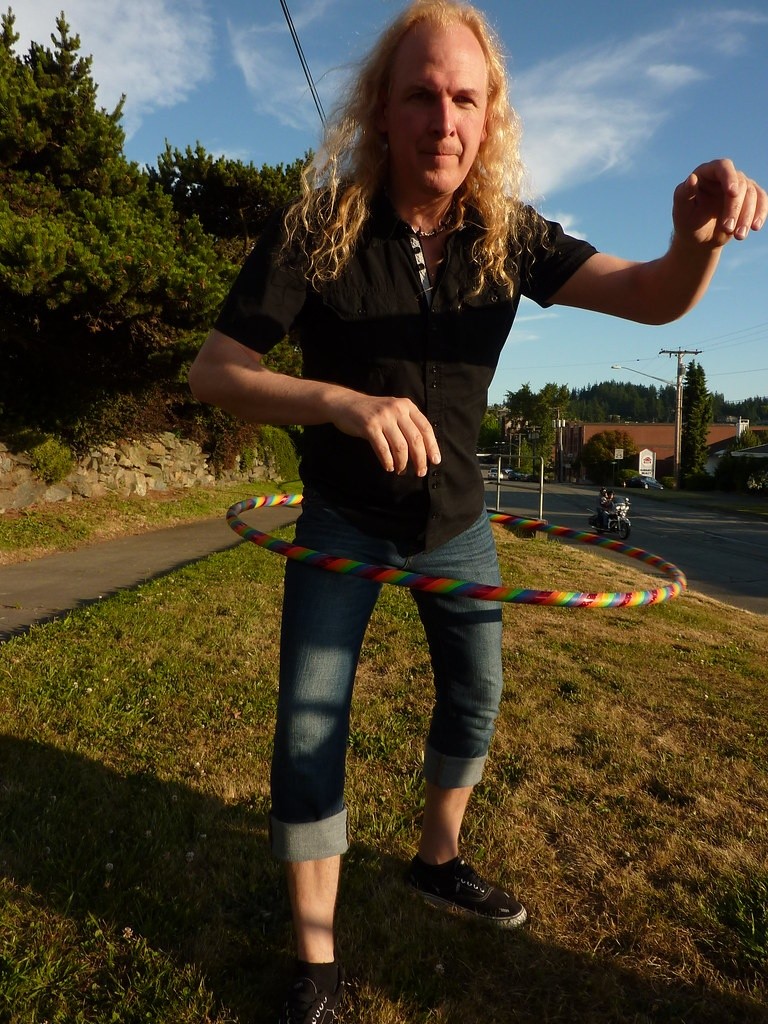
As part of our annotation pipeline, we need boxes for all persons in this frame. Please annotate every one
[597,486,610,530]
[189,0,765,1024]
[603,490,616,530]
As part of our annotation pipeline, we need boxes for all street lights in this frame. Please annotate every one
[495,442,519,449]
[612,365,684,478]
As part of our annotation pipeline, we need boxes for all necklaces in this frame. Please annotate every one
[382,183,457,238]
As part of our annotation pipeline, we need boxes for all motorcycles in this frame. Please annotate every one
[585,497,632,539]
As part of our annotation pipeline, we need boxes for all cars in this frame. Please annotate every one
[624,476,664,490]
[488,468,503,480]
[508,471,521,481]
[502,468,513,474]
[526,475,546,483]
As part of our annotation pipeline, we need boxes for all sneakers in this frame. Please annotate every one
[407,853,527,927]
[271,958,345,1024]
